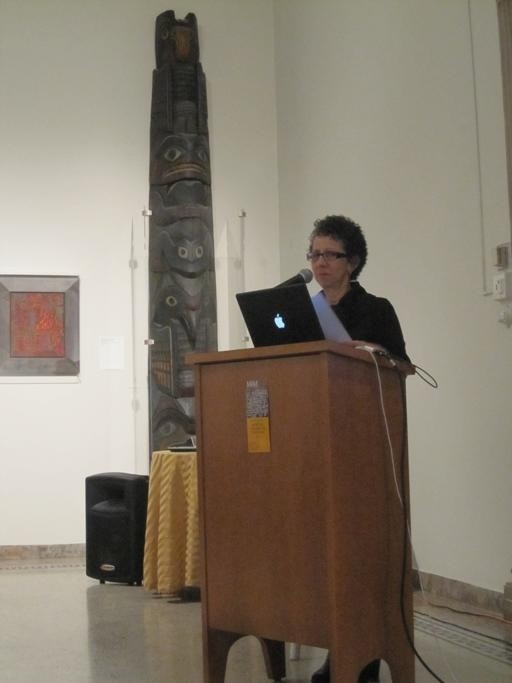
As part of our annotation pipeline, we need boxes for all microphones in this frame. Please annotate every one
[277,270,313,287]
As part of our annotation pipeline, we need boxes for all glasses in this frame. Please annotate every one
[306,248,350,262]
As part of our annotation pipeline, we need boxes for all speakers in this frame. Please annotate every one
[85,473,149,586]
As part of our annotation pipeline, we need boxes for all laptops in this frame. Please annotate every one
[236,285,325,347]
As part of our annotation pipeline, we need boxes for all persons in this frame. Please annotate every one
[306,215,410,683]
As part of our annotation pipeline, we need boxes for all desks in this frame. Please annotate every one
[141,448,202,595]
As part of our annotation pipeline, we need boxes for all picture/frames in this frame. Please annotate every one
[0,275,80,377]
